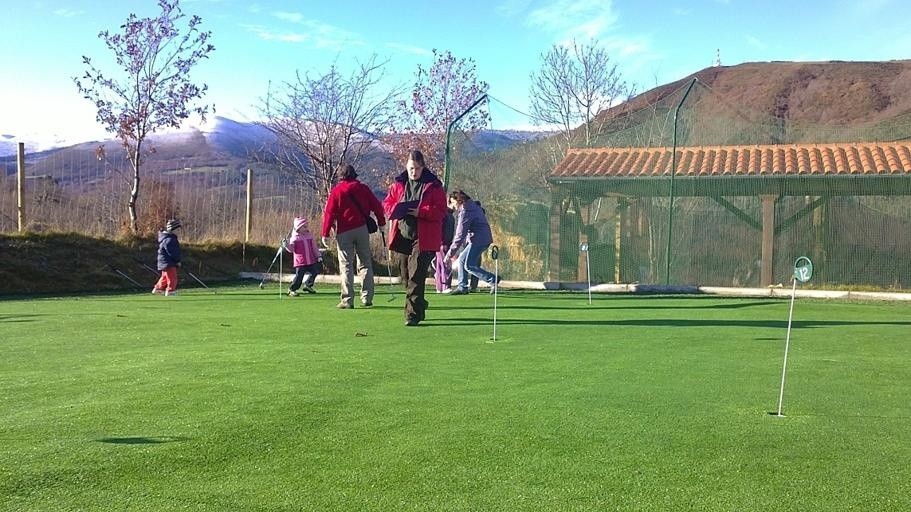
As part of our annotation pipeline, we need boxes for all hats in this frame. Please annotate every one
[294,217,308,231]
[166,220,181,232]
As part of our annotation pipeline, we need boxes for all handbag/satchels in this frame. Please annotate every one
[367,217,377,234]
[442,208,456,244]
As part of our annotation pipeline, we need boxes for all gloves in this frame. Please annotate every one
[318,257,323,263]
[322,237,330,247]
[378,226,385,232]
[282,239,288,247]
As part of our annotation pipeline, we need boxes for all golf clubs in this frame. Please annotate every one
[380,229,396,302]
[259,233,293,289]
[180,264,217,295]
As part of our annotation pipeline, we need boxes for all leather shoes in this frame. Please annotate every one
[489,276,501,295]
[405,300,428,327]
[362,301,372,306]
[337,302,354,309]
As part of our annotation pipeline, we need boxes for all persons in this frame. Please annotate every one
[432,192,505,294]
[151,218,184,296]
[319,164,387,310]
[281,215,324,297]
[380,150,448,327]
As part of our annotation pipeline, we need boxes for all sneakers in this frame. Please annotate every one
[287,284,316,296]
[437,287,477,294]
[152,288,177,296]
